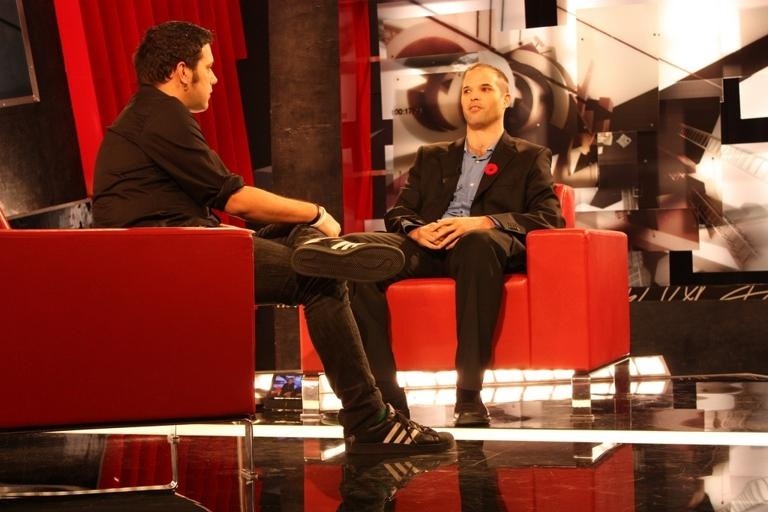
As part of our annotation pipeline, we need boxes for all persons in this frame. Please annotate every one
[91,20,457,454]
[349,61,567,426]
[280,377,295,398]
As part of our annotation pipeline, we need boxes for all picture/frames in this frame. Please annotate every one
[0,0,40,109]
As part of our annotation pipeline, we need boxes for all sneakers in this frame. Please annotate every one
[343,410,454,459]
[287,239,407,284]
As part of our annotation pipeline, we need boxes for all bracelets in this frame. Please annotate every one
[307,202,328,228]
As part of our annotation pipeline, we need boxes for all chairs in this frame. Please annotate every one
[0,201,256,437]
[296,180,631,412]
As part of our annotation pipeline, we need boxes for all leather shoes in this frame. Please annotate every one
[338,386,413,425]
[454,401,493,427]
